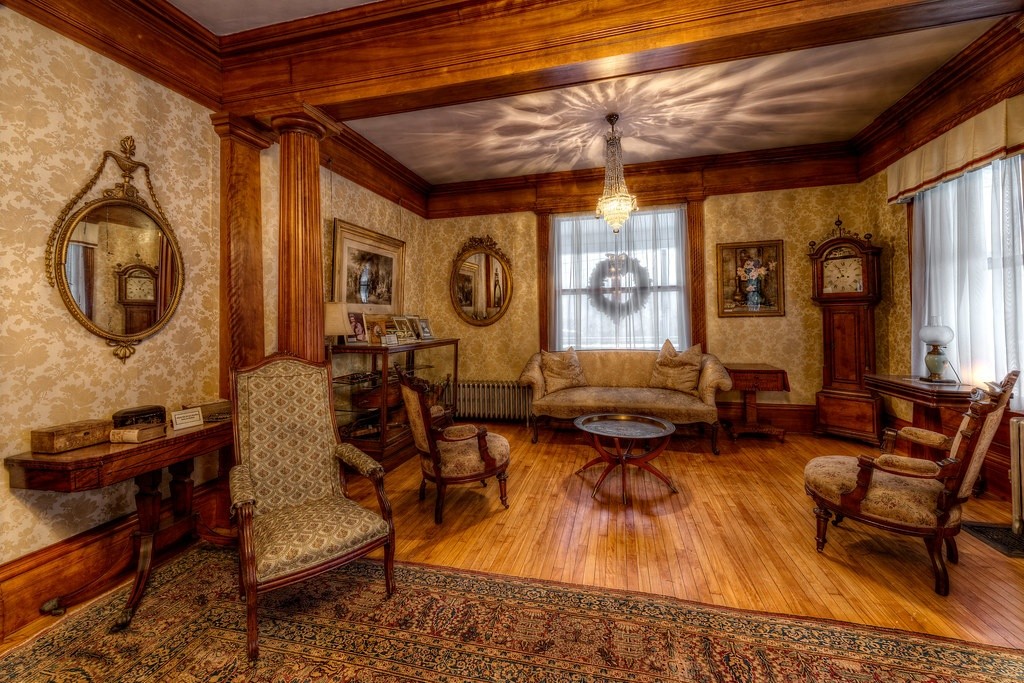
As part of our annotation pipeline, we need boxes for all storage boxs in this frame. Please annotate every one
[112,404,167,429]
[349,425,389,439]
[180,398,231,418]
[30,418,114,455]
[350,385,401,407]
[323,335,345,346]
[346,335,357,342]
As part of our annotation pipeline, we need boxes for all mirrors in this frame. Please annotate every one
[43,135,186,365]
[449,235,515,328]
[493,268,502,307]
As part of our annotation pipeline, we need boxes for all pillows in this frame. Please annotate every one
[540,345,588,397]
[648,339,703,399]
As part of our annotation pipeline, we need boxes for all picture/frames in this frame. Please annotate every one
[456,262,480,316]
[391,317,417,340]
[715,239,786,318]
[386,334,398,344]
[346,311,368,341]
[386,330,407,341]
[367,320,386,343]
[405,315,422,339]
[420,319,433,340]
[331,217,407,317]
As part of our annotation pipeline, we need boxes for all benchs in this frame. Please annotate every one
[518,348,732,456]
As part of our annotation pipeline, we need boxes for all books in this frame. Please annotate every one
[110,424,168,444]
[351,425,389,438]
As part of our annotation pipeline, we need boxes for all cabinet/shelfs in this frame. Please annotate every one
[324,338,461,474]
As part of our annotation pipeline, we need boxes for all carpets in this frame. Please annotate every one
[959,520,1024,560]
[0,538,1024,683]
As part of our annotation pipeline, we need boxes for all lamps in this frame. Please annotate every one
[918,326,957,384]
[595,112,639,235]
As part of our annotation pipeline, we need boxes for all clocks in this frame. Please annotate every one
[115,249,160,334]
[805,215,883,447]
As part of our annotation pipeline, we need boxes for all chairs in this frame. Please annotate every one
[803,370,1021,597]
[393,361,512,526]
[226,349,397,663]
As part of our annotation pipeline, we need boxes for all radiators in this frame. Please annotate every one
[433,378,530,428]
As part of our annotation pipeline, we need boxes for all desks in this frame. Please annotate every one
[721,363,790,446]
[862,373,976,466]
[572,412,679,506]
[4,414,238,636]
